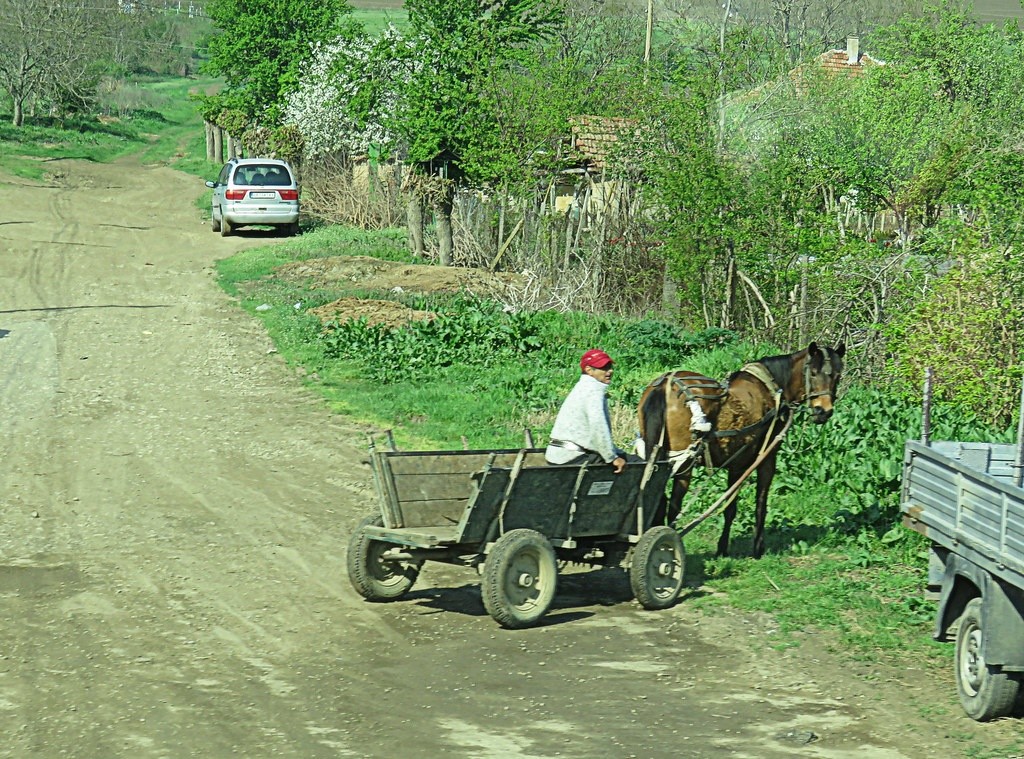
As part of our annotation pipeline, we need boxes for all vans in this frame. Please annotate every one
[205,157,300,237]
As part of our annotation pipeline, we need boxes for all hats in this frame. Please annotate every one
[580,349,616,372]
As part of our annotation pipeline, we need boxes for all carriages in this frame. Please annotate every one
[346,341,849,631]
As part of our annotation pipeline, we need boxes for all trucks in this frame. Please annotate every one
[899,436,1024,721]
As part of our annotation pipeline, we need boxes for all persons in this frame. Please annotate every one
[544,349,644,474]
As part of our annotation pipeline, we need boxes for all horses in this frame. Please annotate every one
[638,341,846,560]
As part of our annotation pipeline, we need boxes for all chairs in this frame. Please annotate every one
[264,172,278,185]
[250,173,264,186]
[235,172,248,185]
